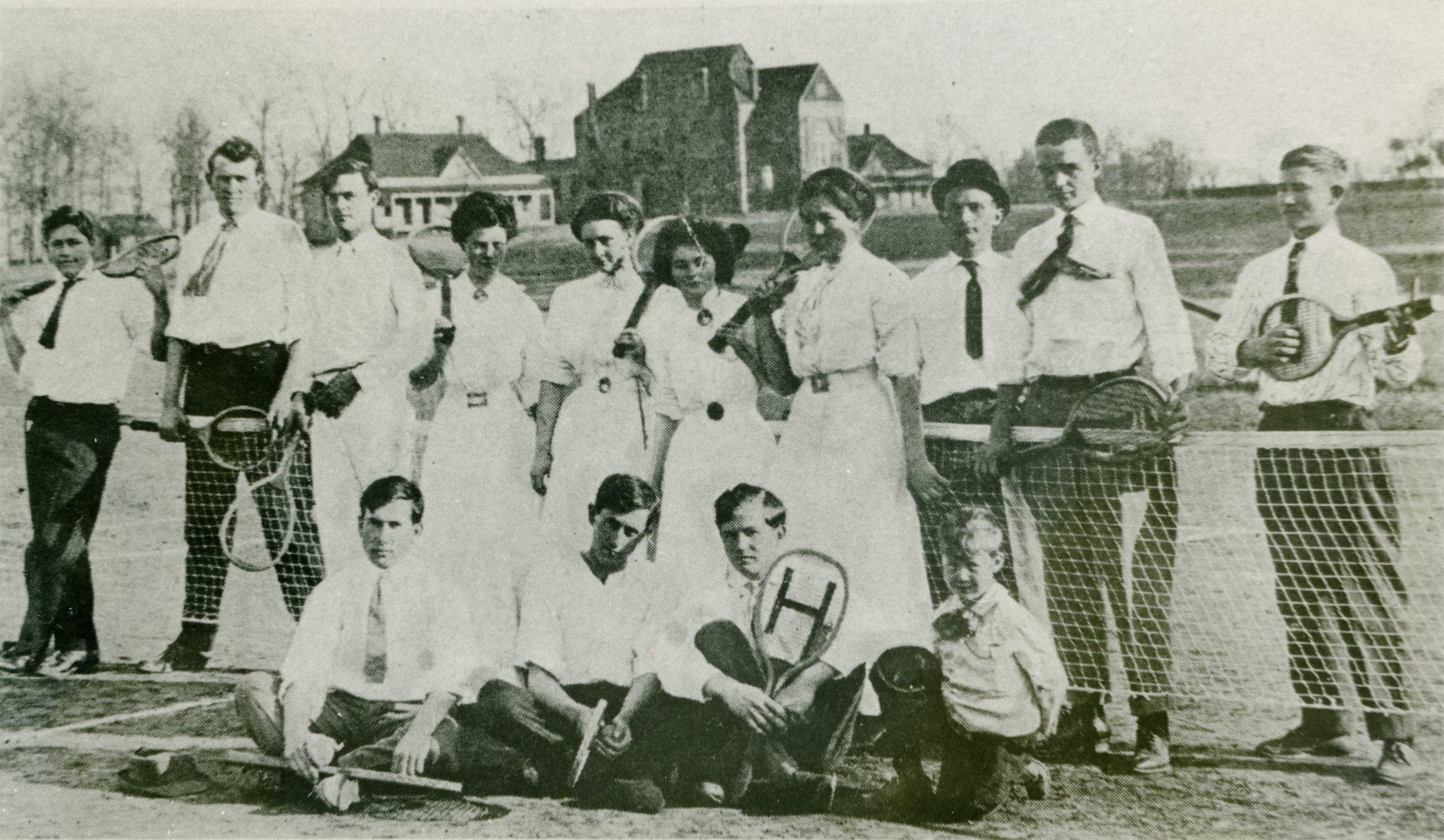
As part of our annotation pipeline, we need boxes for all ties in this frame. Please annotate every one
[1019,216,1079,304]
[182,218,238,296]
[960,259,983,360]
[364,581,387,686]
[1281,240,1306,365]
[37,277,82,349]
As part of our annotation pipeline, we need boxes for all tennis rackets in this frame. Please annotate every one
[996,376,1168,472]
[218,381,327,572]
[613,213,680,358]
[1256,295,1444,381]
[8,234,182,304]
[706,206,813,355]
[745,209,877,320]
[224,749,462,796]
[129,406,277,472]
[566,699,609,791]
[408,223,468,343]
[727,548,849,807]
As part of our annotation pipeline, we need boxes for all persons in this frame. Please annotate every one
[1205,145,1429,787]
[159,137,1067,825]
[0,204,171,679]
[972,117,1198,777]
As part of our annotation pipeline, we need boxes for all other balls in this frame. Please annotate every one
[304,733,336,767]
[601,723,622,742]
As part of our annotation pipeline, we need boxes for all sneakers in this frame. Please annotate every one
[1258,722,1355,758]
[1375,738,1422,784]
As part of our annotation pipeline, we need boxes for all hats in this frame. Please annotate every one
[116,747,210,798]
[931,158,1010,215]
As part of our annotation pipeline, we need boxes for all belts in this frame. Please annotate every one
[1264,400,1362,419]
[1033,366,1135,392]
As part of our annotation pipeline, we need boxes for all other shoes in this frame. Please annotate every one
[139,643,208,671]
[0,641,37,673]
[39,648,98,681]
[786,770,837,812]
[601,776,666,814]
[1131,715,1170,774]
[1015,754,1052,801]
[1041,704,1110,753]
[693,776,732,807]
[313,773,363,812]
[875,770,936,806]
